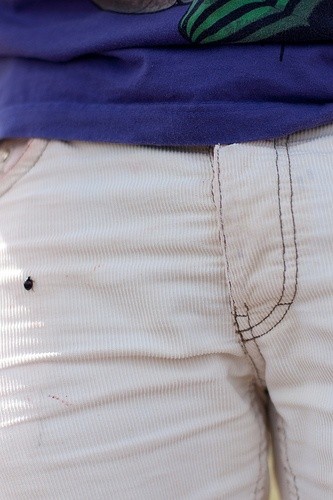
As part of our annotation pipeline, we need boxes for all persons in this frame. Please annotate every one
[0,0,333,499]
